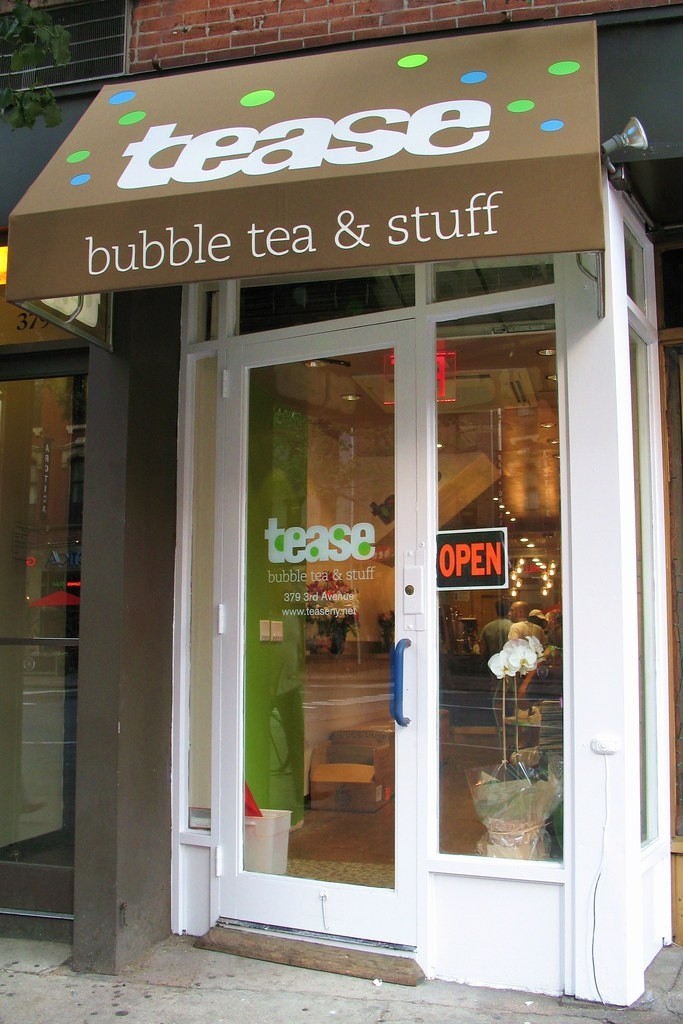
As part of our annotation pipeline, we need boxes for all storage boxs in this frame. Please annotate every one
[307,740,394,813]
[245,808,292,875]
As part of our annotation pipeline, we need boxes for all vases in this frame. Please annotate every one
[318,616,348,657]
[382,635,394,652]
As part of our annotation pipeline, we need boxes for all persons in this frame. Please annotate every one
[507,601,561,647]
[480,600,512,727]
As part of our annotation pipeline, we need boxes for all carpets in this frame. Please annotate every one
[287,858,395,888]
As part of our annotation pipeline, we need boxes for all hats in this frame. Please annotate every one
[529,610,545,619]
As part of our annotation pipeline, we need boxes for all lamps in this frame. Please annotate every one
[509,560,557,598]
[600,117,650,173]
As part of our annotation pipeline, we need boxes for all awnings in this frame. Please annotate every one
[5,21,606,353]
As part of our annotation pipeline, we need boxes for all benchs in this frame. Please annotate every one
[330,708,451,766]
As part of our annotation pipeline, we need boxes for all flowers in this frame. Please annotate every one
[487,637,544,762]
[379,611,395,636]
[308,574,361,638]
